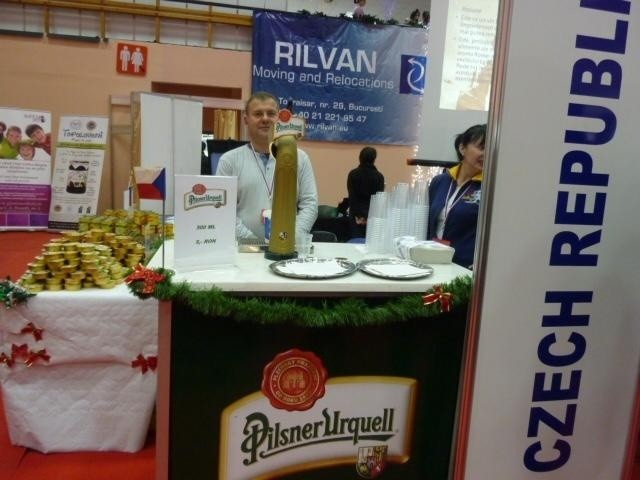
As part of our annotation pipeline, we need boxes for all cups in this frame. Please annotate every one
[263,210,272,241]
[364,178,429,254]
[295,233,314,258]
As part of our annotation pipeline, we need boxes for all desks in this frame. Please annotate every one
[145,239,473,480]
[0,270,160,455]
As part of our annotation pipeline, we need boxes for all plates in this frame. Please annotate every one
[270,257,356,279]
[357,258,433,279]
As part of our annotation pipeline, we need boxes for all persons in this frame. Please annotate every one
[1,125,34,159]
[213,89,319,258]
[343,143,387,243]
[18,142,35,161]
[202,141,209,175]
[0,121,8,139]
[26,123,52,154]
[424,120,488,271]
